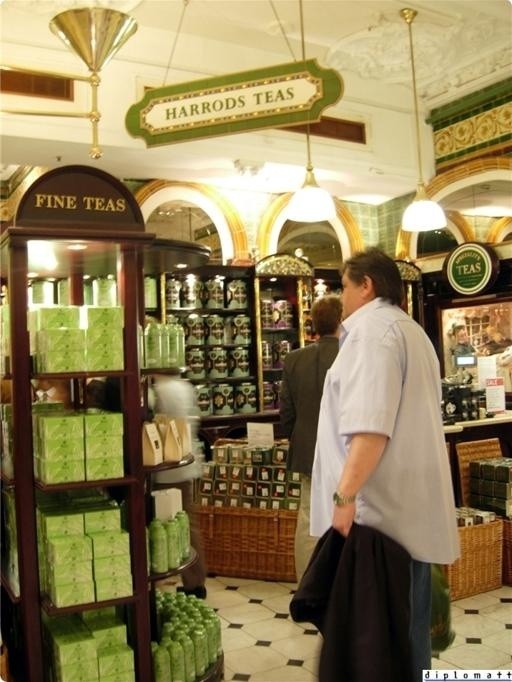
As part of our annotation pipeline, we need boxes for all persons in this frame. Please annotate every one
[271,296,348,588]
[149,375,209,601]
[451,322,479,370]
[291,247,462,681]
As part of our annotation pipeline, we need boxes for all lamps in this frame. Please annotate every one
[0,3,140,159]
[284,1,337,225]
[400,7,449,235]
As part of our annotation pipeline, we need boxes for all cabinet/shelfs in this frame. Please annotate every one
[0,157,316,681]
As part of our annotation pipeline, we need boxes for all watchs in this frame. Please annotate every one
[331,491,358,507]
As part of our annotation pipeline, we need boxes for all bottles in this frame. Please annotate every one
[146,322,187,367]
[147,510,192,575]
[151,586,222,680]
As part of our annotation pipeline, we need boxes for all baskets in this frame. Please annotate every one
[188,506,298,582]
[441,437,512,602]
